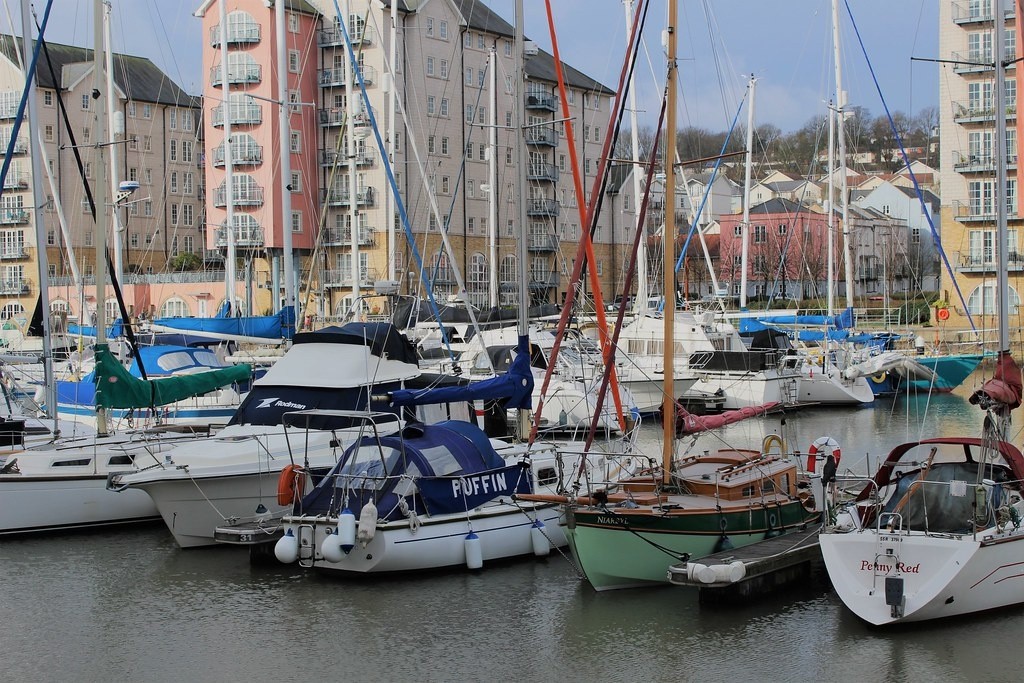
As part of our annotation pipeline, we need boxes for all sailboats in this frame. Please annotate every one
[0,1,1023,633]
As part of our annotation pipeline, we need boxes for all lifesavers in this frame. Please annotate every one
[938,309,950,321]
[806,348,825,366]
[806,436,841,474]
[277,463,308,507]
[871,370,887,384]
